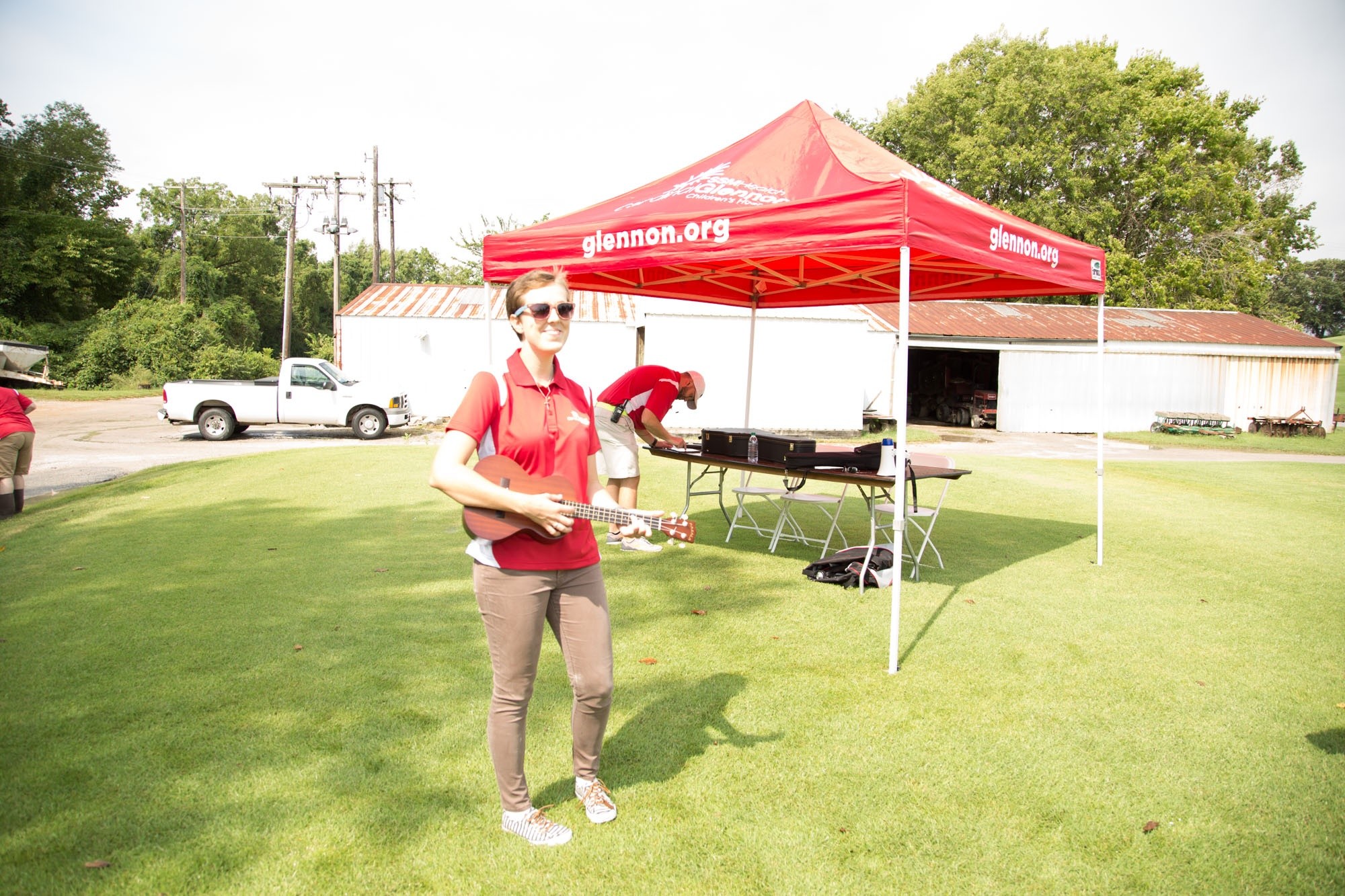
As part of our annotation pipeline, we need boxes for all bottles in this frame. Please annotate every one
[747,432,758,464]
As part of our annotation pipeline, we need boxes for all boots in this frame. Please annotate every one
[0,494,15,520]
[13,489,25,510]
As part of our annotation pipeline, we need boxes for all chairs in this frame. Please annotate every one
[868,452,956,580]
[724,446,853,560]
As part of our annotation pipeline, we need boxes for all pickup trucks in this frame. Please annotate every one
[157,357,412,442]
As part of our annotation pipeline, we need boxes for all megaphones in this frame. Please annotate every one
[877,439,912,477]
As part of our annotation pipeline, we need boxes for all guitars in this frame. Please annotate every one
[461,454,698,551]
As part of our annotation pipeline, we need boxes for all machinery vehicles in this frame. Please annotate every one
[908,349,997,429]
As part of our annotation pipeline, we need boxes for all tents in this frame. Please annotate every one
[479,100,1115,677]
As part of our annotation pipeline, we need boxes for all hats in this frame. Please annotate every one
[685,371,705,409]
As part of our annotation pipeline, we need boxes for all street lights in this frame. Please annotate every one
[313,215,359,368]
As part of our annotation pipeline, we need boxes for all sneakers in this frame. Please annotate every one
[620,535,663,553]
[502,804,573,848]
[575,777,618,824]
[606,530,623,544]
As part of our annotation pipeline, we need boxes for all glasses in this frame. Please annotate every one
[515,302,576,320]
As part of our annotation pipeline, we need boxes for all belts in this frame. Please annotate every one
[596,401,628,416]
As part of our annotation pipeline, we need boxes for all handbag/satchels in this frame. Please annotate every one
[803,540,894,589]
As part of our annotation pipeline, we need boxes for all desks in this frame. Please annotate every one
[642,445,973,595]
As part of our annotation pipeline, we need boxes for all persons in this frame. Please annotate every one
[1,384,38,524]
[430,270,653,850]
[591,364,705,551]
